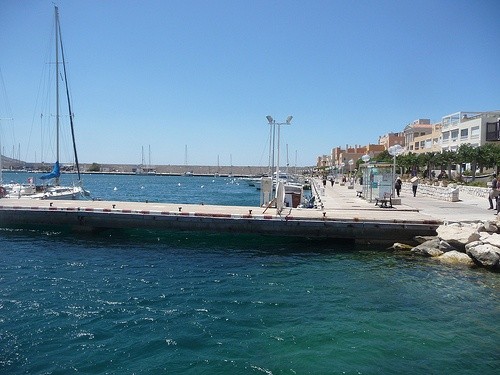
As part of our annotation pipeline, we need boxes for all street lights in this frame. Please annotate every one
[341,163,345,183]
[388,145,404,198]
[266,115,293,207]
[362,155,371,197]
[348,159,355,186]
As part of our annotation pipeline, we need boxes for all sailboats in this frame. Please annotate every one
[215,155,221,178]
[135,143,156,176]
[228,153,234,178]
[182,144,194,177]
[242,118,312,191]
[0,4,84,202]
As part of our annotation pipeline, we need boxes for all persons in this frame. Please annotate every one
[342,173,364,187]
[412,179,418,198]
[322,178,326,188]
[395,177,402,197]
[329,176,334,188]
[493,176,500,216]
[487,173,498,210]
